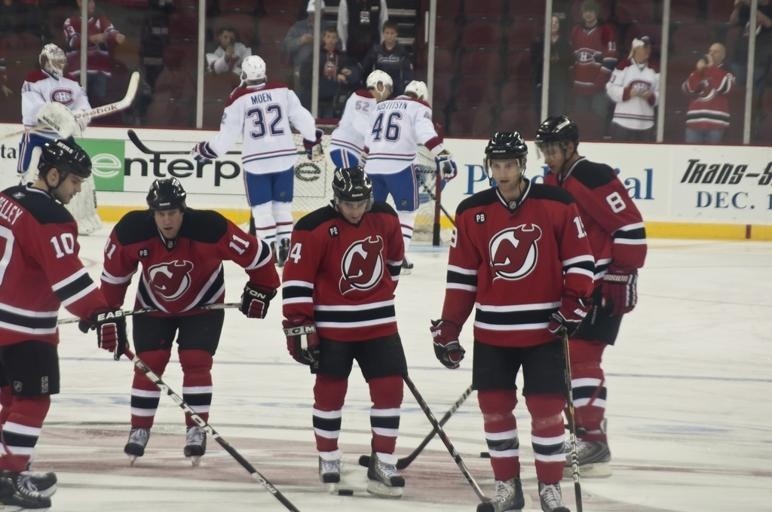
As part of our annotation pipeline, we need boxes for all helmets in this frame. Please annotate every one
[38,43,67,77]
[331,168,373,201]
[365,69,394,94]
[36,139,92,181]
[485,129,528,159]
[535,112,579,152]
[145,177,187,210]
[404,79,428,103]
[239,55,268,83]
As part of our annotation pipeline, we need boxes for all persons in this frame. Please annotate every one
[283,1,417,116]
[532,114,648,469]
[326,69,394,169]
[531,1,660,143]
[0,139,127,510]
[728,0,771,36]
[77,175,280,458]
[62,2,251,126]
[360,79,457,271]
[15,41,103,238]
[430,128,597,511]
[680,42,736,143]
[194,53,321,265]
[282,168,408,488]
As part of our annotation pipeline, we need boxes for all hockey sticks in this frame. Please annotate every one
[564,329,582,512]
[1,71,140,141]
[404,375,492,501]
[127,129,306,155]
[358,380,474,469]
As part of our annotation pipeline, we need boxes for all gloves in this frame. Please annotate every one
[280,318,322,366]
[40,102,79,136]
[301,128,326,163]
[594,272,639,320]
[90,307,131,363]
[239,280,277,319]
[545,296,593,339]
[430,320,465,370]
[434,150,458,183]
[78,316,98,335]
[190,140,219,162]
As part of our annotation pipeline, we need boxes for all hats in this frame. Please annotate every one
[628,34,653,48]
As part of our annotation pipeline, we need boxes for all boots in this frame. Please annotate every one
[317,454,342,483]
[23,471,58,493]
[271,237,291,268]
[366,450,407,488]
[0,469,52,509]
[476,475,525,512]
[124,426,151,457]
[183,426,207,457]
[537,480,571,512]
[564,435,614,469]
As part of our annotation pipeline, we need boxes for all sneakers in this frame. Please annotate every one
[401,257,415,274]
